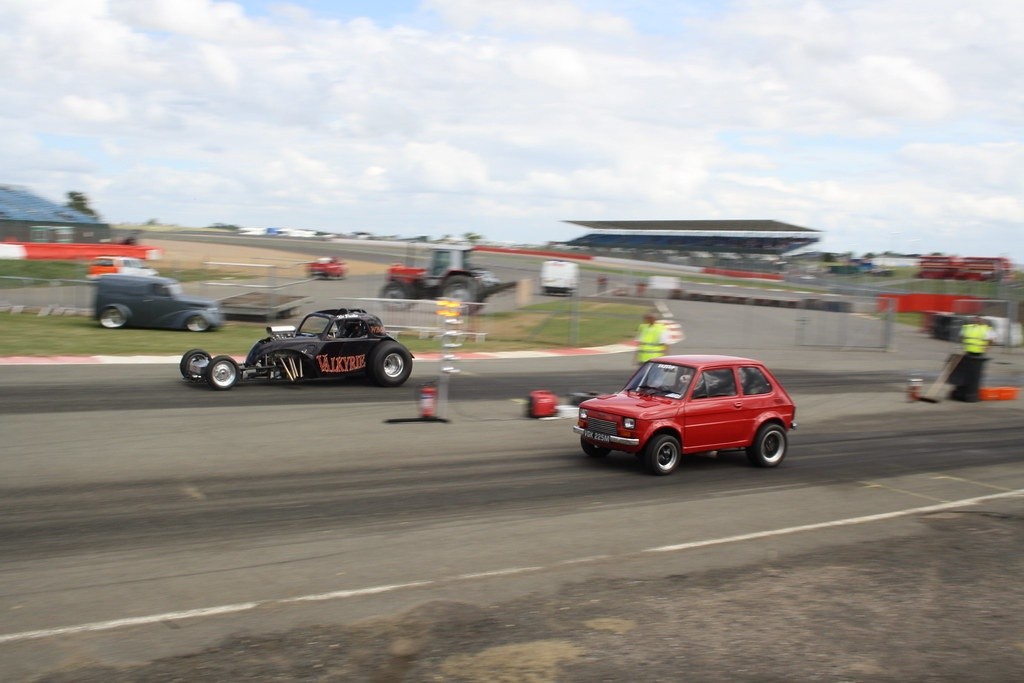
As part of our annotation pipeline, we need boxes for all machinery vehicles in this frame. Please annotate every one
[379,243,519,322]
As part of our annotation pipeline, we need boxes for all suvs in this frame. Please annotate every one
[573,354,797,477]
[91,272,224,332]
[85,255,159,284]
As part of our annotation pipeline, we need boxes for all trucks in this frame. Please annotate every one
[538,259,580,300]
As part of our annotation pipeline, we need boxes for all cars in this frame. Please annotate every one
[180,306,415,391]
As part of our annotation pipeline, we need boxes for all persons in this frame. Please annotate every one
[957,315,997,400]
[633,314,667,369]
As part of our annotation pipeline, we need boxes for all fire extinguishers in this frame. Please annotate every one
[420,380,438,419]
